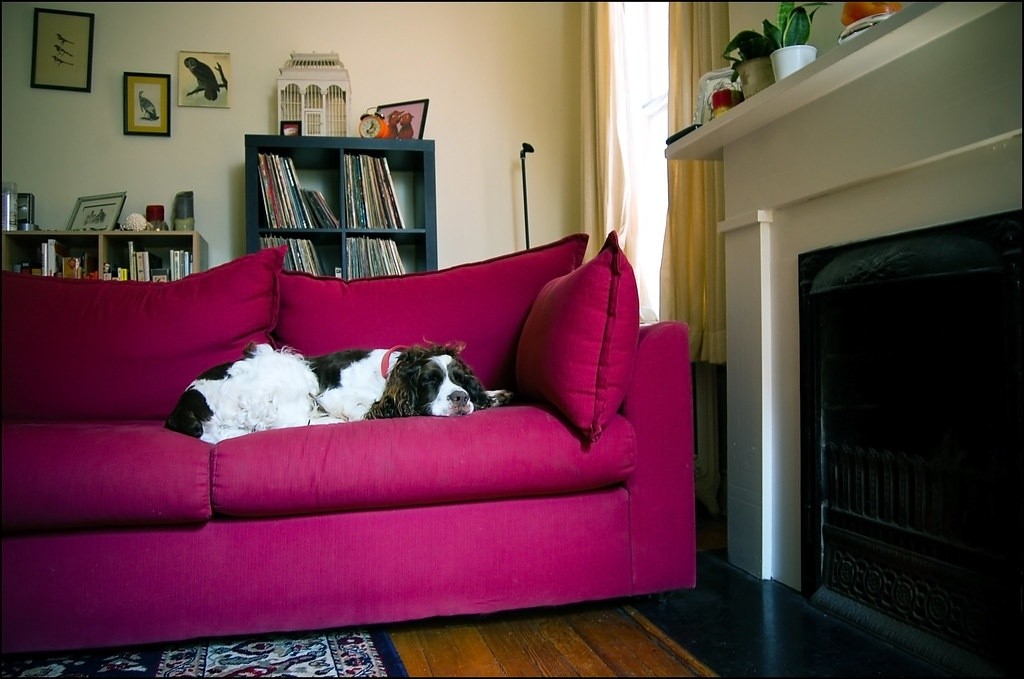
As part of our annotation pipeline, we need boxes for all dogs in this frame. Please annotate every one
[164,342,513,446]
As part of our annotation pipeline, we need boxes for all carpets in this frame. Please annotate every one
[0,624,409,679]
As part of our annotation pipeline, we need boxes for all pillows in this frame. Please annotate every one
[275,233,589,391]
[0,244,289,420]
[513,231,641,442]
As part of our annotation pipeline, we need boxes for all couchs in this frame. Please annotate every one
[0,320,696,650]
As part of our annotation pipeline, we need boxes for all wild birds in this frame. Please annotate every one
[52,34,73,66]
[139,91,157,120]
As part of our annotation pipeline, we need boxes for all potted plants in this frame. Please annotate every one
[721,2,832,101]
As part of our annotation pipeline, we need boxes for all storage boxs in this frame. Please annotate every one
[151,269,170,284]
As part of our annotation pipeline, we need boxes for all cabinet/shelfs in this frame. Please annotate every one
[2,230,211,281]
[244,134,438,281]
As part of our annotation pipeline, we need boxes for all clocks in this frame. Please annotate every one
[359,107,388,139]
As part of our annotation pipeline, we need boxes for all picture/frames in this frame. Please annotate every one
[280,121,301,136]
[693,66,742,128]
[178,50,230,108]
[377,99,430,140]
[65,191,127,232]
[124,71,171,137]
[30,8,95,93]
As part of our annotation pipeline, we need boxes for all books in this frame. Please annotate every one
[345,236,406,281]
[342,153,406,229]
[258,236,324,277]
[103,240,193,283]
[256,153,339,229]
